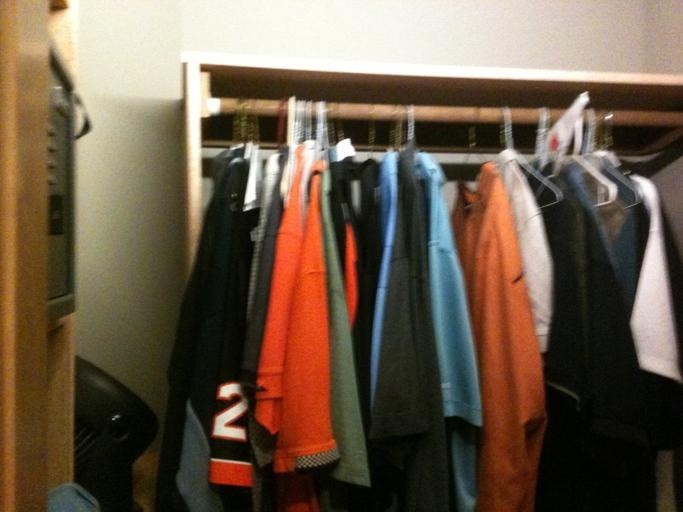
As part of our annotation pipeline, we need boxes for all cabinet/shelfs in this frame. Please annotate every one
[0,1,75,512]
